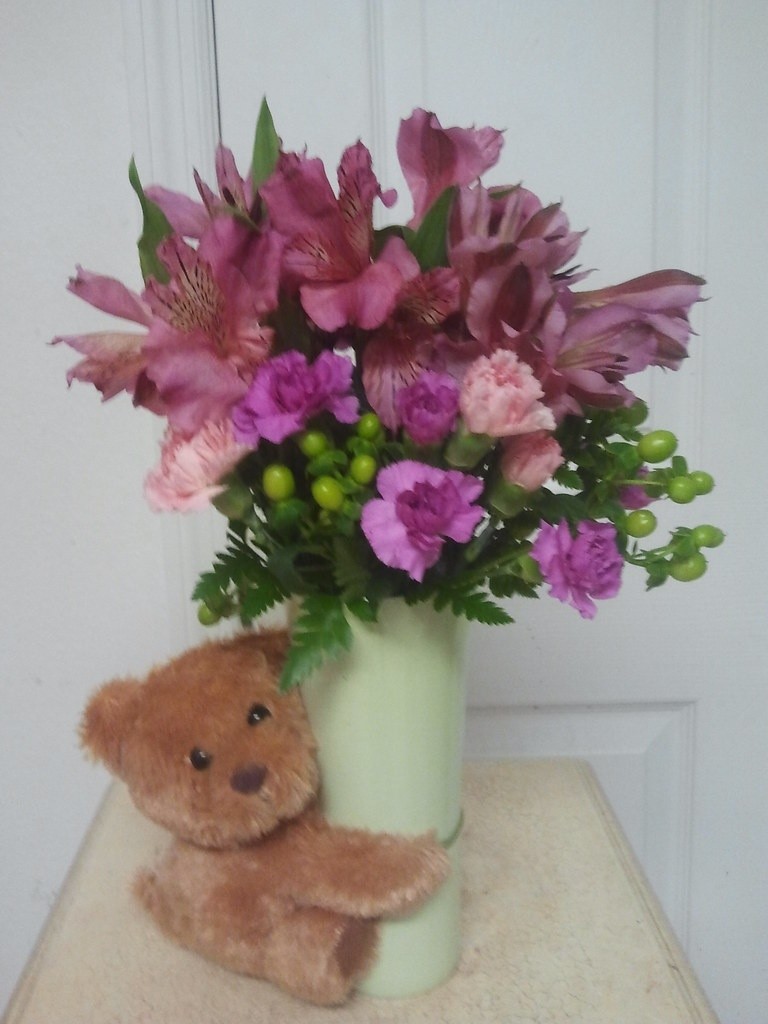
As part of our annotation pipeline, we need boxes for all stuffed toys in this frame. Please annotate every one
[78,625,450,1009]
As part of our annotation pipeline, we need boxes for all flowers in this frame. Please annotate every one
[46,93,719,695]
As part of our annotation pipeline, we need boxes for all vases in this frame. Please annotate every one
[287,586,480,998]
